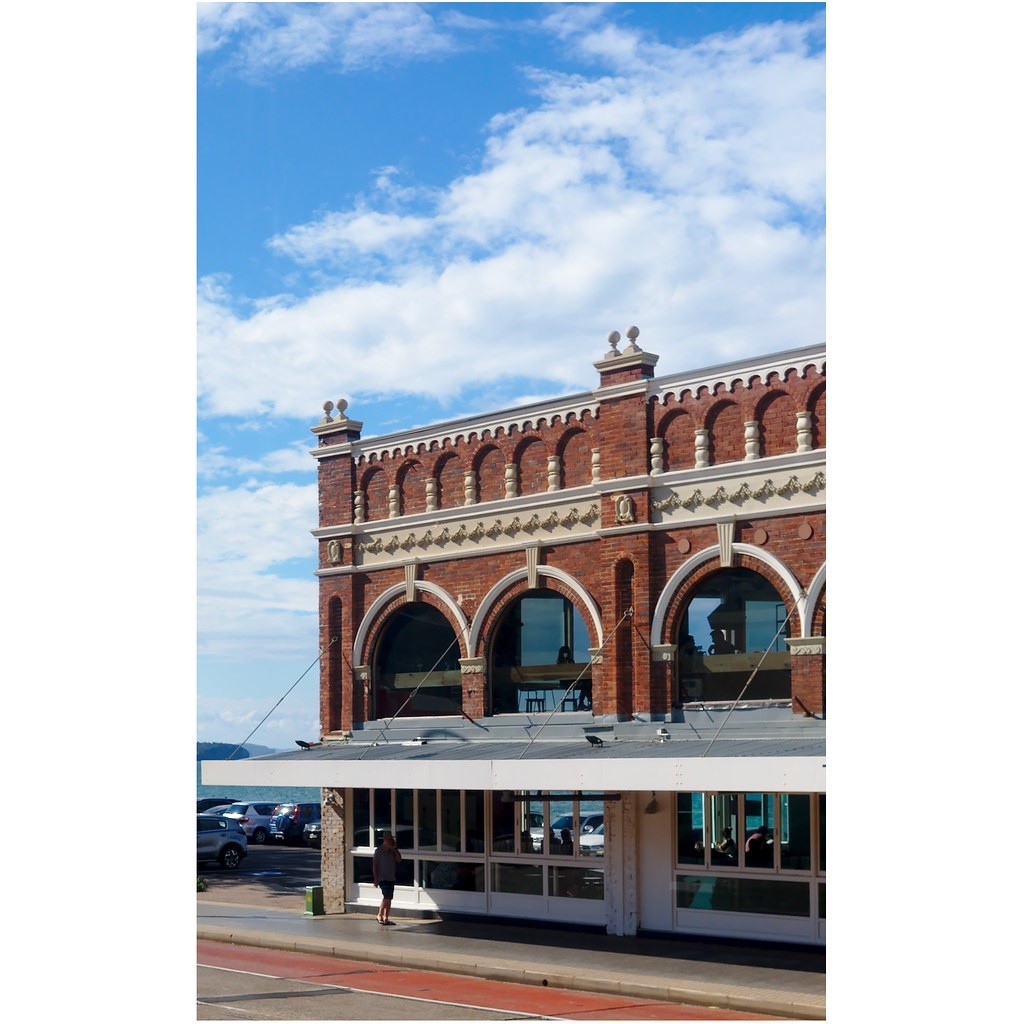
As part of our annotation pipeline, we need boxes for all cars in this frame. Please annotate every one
[197,812,248,869]
[551,810,604,848]
[223,800,285,845]
[197,798,241,814]
[206,803,231,816]
[529,811,551,844]
[302,818,321,849]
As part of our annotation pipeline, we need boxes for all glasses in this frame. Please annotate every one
[389,839,394,841]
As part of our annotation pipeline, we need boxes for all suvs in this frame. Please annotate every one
[268,802,321,847]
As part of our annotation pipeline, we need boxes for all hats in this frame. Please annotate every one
[384,834,396,839]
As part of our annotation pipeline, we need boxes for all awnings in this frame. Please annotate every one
[201,742,780,792]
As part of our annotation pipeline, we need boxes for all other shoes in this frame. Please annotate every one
[383,921,395,925]
[376,918,383,924]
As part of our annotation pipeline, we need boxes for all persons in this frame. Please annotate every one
[372,834,401,926]
[541,827,573,855]
[557,645,592,709]
[715,826,768,850]
[687,635,706,654]
[708,630,735,655]
